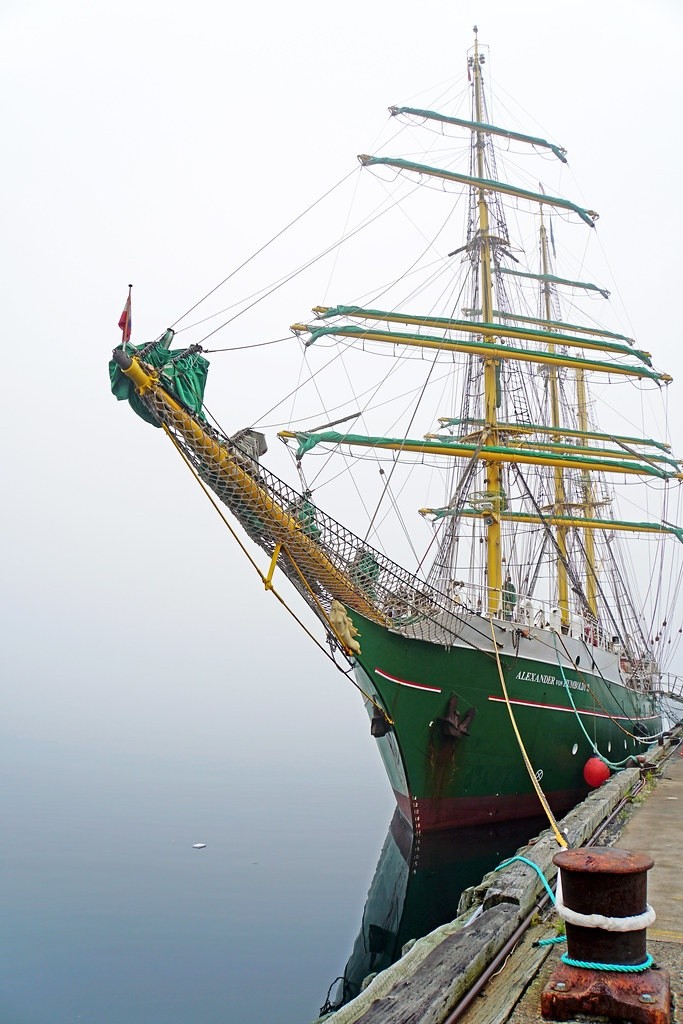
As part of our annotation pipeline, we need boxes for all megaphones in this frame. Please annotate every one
[485,517,493,525]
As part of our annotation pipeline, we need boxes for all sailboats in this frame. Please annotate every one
[109,24,683,834]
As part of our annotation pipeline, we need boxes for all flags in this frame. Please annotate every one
[118,284,133,343]
